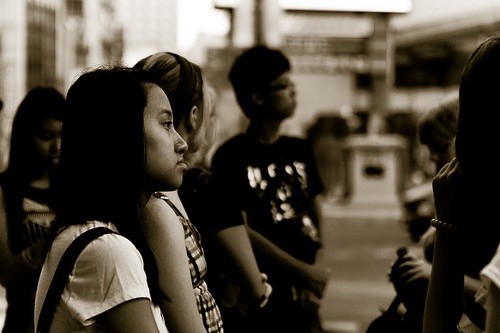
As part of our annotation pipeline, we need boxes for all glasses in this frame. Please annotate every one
[264,83,295,94]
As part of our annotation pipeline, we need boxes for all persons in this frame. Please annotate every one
[0,46,334,333]
[366,34,500,333]
